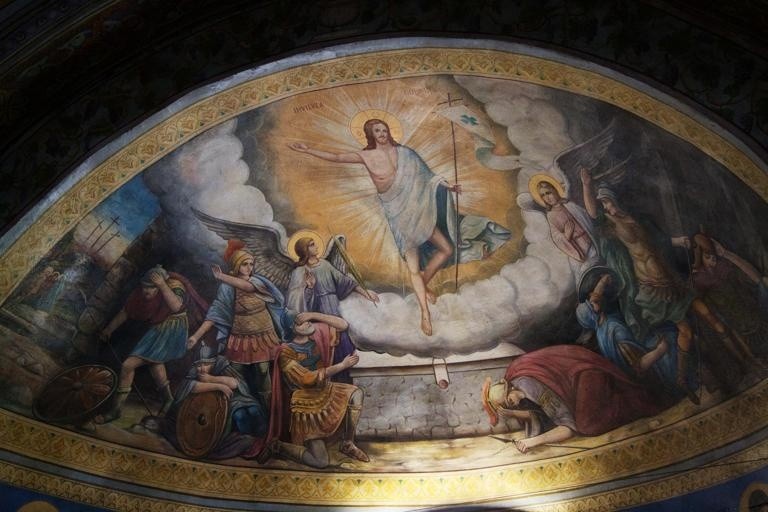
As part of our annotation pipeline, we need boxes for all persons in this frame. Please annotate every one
[481,344,659,454]
[163,342,263,461]
[286,118,461,336]
[95,264,204,426]
[242,312,370,468]
[286,237,380,385]
[186,239,284,417]
